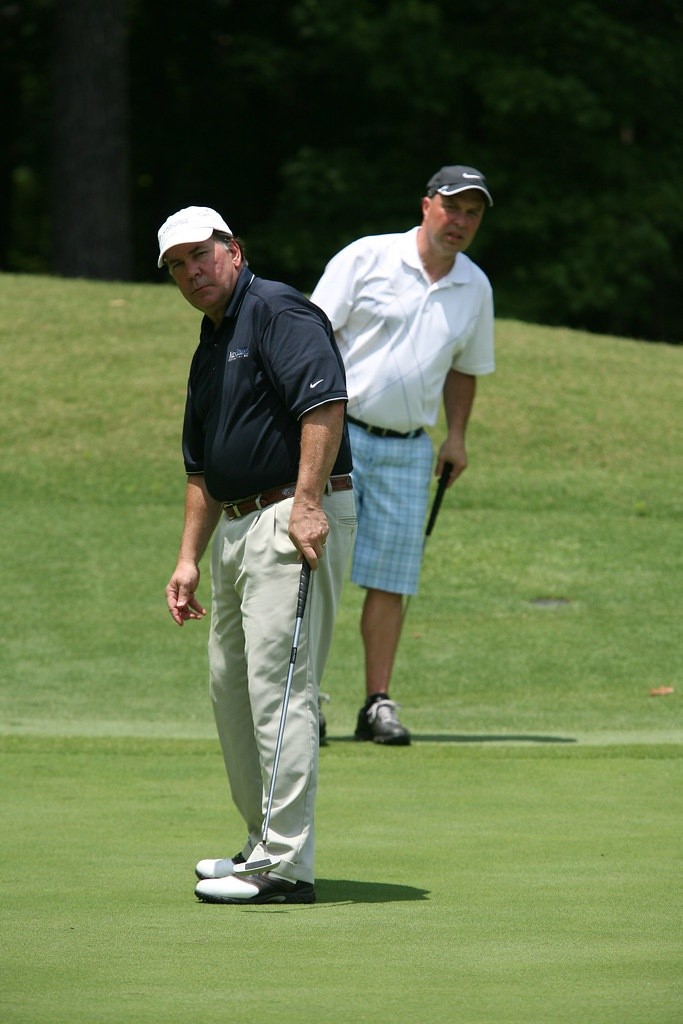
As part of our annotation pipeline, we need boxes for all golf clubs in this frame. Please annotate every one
[403,459,452,612]
[232,552,312,875]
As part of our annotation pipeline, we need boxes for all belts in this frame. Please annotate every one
[346,414,423,439]
[221,475,353,520]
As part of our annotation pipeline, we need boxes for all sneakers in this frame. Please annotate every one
[354,694,410,746]
[194,852,248,880]
[317,693,330,744]
[194,870,316,904]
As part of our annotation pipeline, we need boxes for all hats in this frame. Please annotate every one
[427,166,493,207]
[158,206,233,269]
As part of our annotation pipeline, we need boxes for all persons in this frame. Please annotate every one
[310,166,495,745]
[158,207,357,905]
